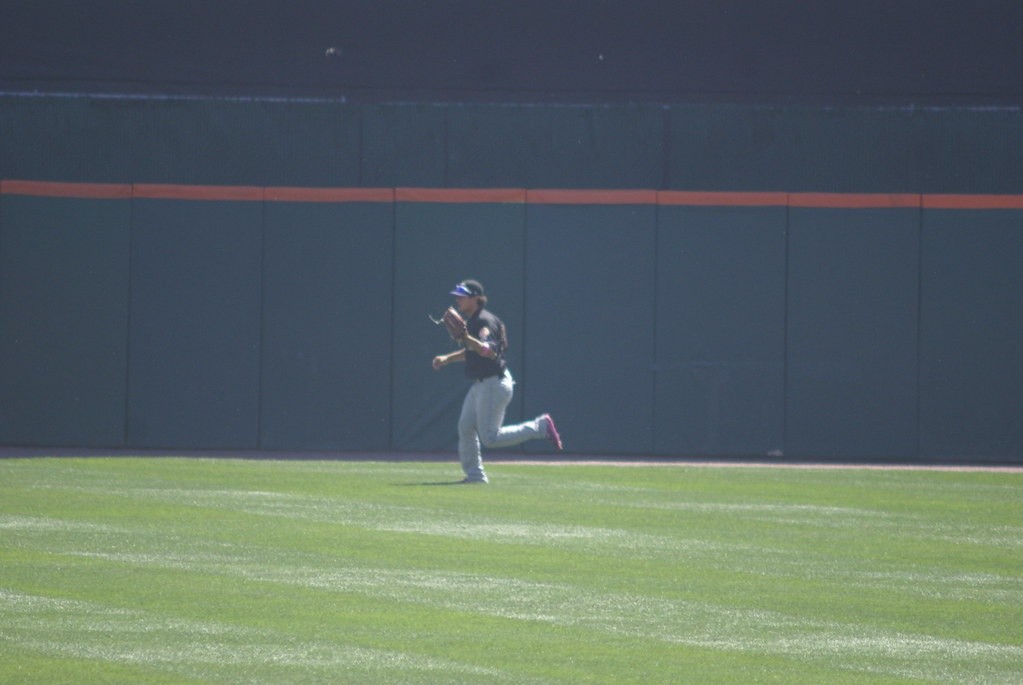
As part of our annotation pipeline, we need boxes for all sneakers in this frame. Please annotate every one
[541,413,563,450]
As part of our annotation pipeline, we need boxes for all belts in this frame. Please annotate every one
[480,373,505,383]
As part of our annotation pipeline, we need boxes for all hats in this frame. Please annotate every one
[450,279,484,297]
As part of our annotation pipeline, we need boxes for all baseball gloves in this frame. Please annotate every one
[443,307,467,344]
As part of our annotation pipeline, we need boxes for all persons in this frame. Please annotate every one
[432,280,563,483]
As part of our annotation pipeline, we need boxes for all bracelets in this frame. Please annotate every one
[479,343,490,357]
